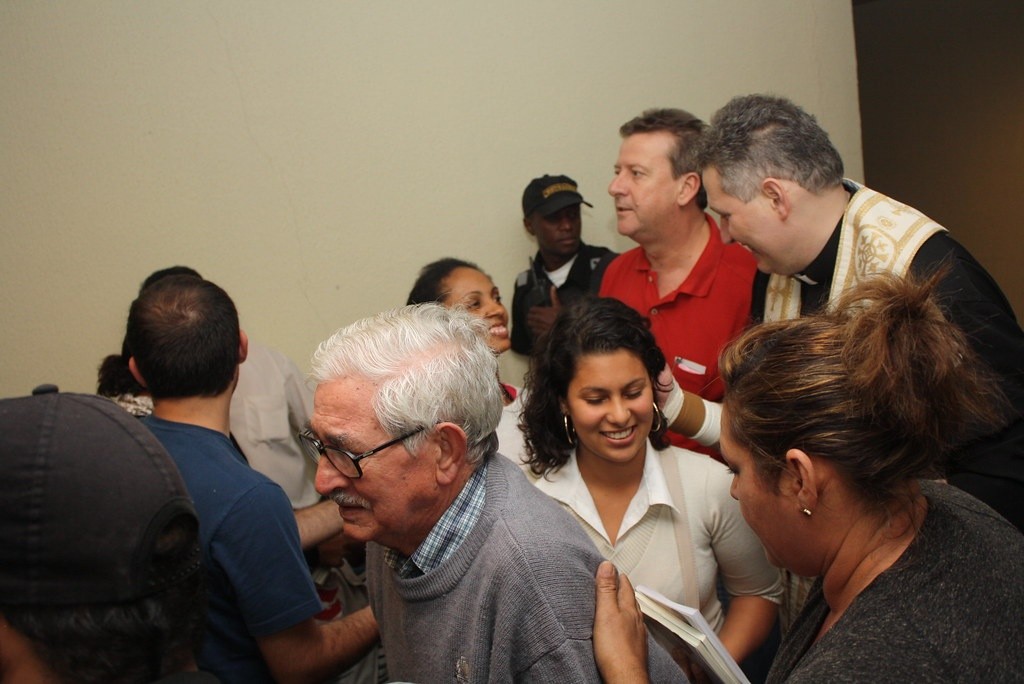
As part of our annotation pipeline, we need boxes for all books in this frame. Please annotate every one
[634,586,751,684]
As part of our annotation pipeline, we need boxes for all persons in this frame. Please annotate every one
[509,175,622,358]
[718,278,1024,684]
[652,94,1024,640]
[519,296,784,684]
[300,304,689,684]
[598,108,758,464]
[97,274,378,684]
[139,265,322,508]
[407,258,545,485]
[0,386,222,684]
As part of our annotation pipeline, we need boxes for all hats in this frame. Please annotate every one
[0,383,202,612]
[523,173,593,218]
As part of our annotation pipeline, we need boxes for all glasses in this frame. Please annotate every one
[297,422,441,477]
